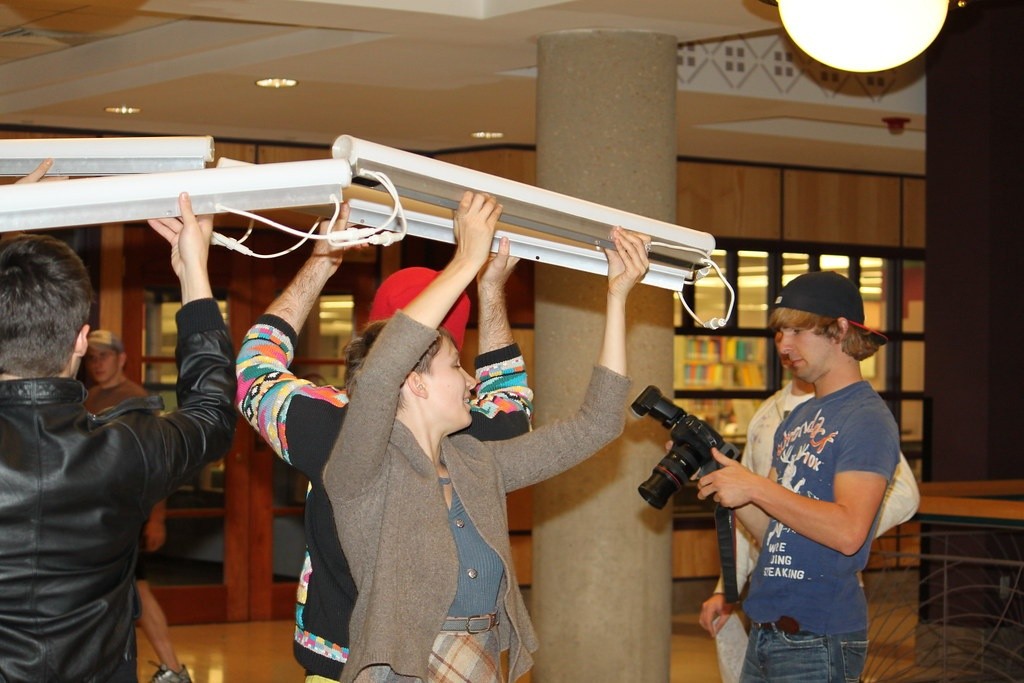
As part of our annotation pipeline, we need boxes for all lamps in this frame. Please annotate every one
[0,160,408,248]
[331,135,716,284]
[778,0,951,73]
[0,135,216,175]
[216,157,735,330]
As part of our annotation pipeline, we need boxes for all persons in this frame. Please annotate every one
[234,202,534,682]
[700,316,920,681]
[80,329,190,682]
[323,190,650,683]
[1,159,237,682]
[694,271,901,682]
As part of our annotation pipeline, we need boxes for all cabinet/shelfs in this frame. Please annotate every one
[673,333,792,443]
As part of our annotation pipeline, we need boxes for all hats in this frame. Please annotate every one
[773,270,889,346]
[87,329,123,353]
[369,267,471,351]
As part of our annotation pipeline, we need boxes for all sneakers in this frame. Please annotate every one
[146,659,191,683]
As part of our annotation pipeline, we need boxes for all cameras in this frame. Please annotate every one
[628,384,743,510]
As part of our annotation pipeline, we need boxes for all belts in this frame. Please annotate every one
[440,608,497,634]
[754,612,800,633]
